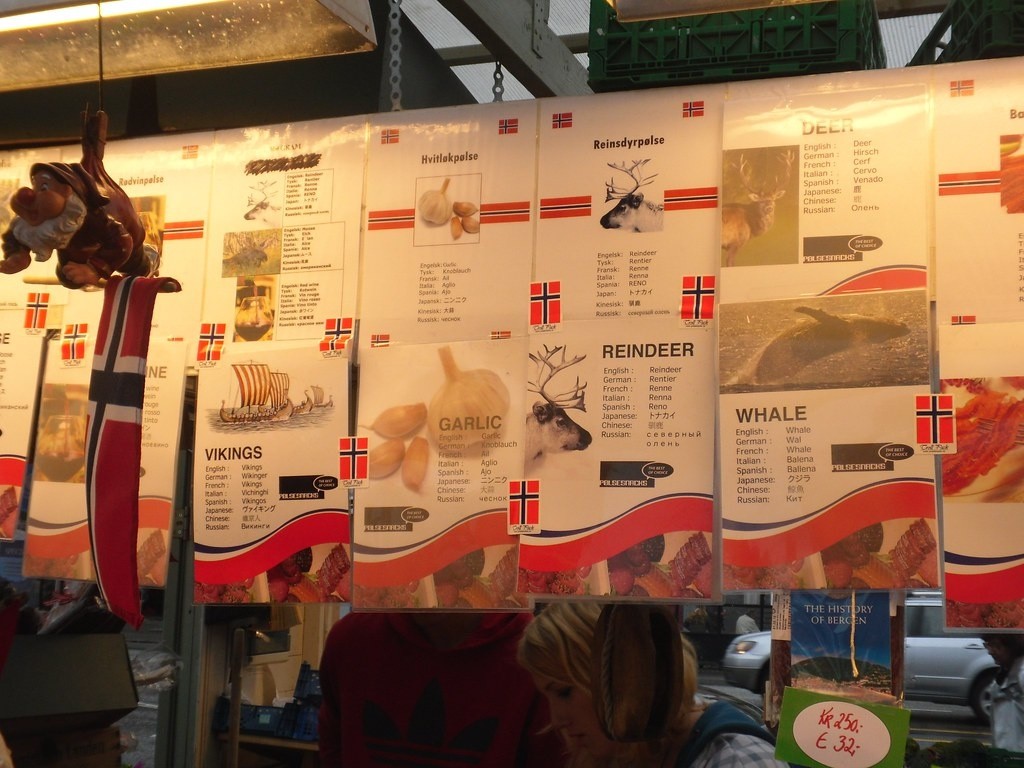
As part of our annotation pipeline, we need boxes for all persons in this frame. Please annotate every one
[976,633,1024,756]
[0,575,24,674]
[319,606,559,768]
[695,605,727,636]
[517,599,791,768]
[735,606,760,635]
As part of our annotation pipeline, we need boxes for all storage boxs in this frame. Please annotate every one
[5,726,123,768]
[0,635,141,736]
[586,0,887,94]
[904,0,1024,67]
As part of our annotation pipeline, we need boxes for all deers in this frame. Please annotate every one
[600,159,664,233]
[525,344,592,479]
[244,180,282,223]
[721,150,796,268]
[222,233,276,270]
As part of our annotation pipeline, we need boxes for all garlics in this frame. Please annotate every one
[417,177,479,242]
[361,344,511,492]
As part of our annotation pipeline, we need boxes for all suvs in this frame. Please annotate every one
[722,591,1001,723]
